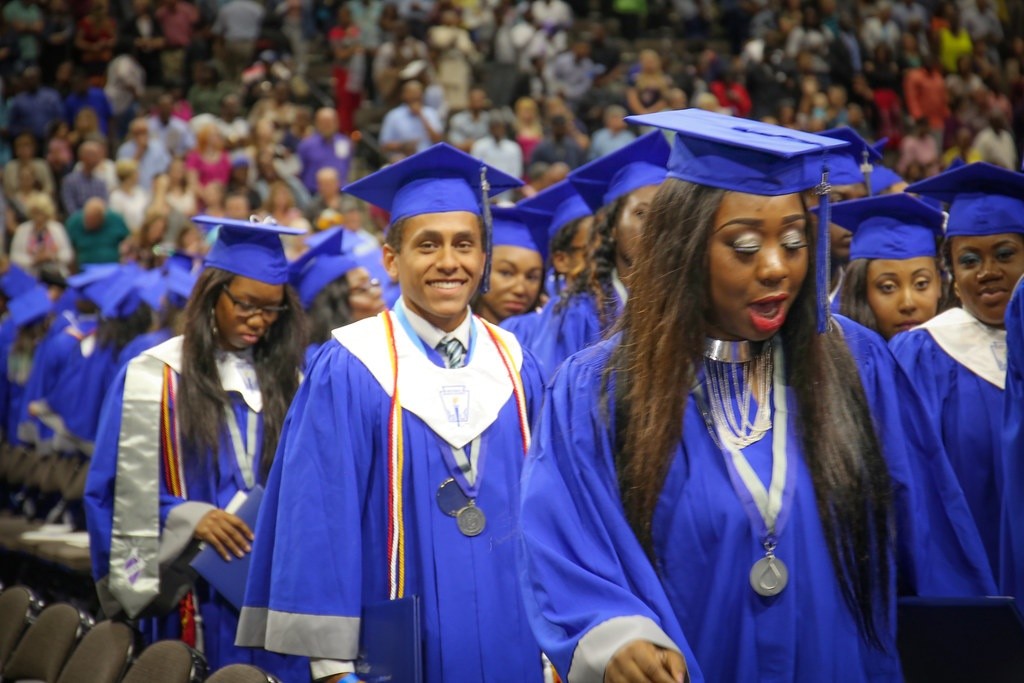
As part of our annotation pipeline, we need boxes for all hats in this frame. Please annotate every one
[289,230,356,308]
[189,213,306,285]
[490,205,553,257]
[810,127,886,186]
[340,142,529,294]
[905,161,1024,237]
[806,194,945,260]
[4,261,193,330]
[566,129,673,203]
[622,107,850,332]
[516,177,596,240]
[863,137,904,194]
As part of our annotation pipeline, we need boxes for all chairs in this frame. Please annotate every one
[0,444,281,683]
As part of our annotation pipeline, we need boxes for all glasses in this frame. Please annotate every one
[222,285,280,319]
[345,278,379,296]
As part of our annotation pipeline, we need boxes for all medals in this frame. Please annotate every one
[749,557,790,598]
[434,473,486,537]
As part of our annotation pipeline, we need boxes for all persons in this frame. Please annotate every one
[64,129,675,683]
[0,0,1023,461]
[801,119,1023,609]
[512,109,998,683]
